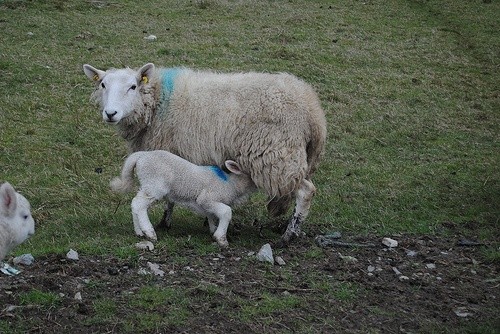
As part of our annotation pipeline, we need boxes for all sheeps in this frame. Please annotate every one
[1,182,35,267]
[109,149,257,248]
[83,62,326,246]
